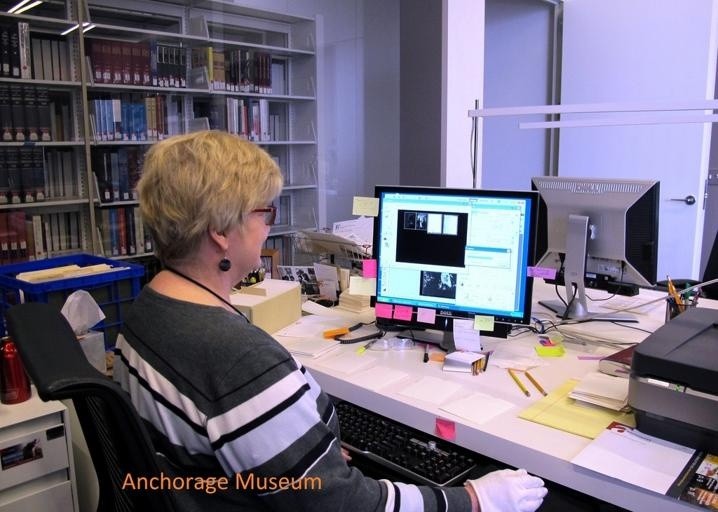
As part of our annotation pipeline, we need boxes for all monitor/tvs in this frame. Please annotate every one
[532,178,659,323]
[373,185,539,355]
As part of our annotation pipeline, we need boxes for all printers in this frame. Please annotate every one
[630,307,717,458]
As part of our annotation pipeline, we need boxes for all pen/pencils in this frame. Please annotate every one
[483,351,490,373]
[423,342,430,363]
[471,357,483,377]
[525,369,549,397]
[508,367,532,398]
[665,274,684,314]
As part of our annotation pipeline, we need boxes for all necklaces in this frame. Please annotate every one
[166,262,253,327]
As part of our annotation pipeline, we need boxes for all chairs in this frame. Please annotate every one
[4,304,176,512]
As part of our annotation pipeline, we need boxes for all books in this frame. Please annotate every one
[1,23,68,80]
[85,40,186,88]
[197,98,285,141]
[91,93,184,142]
[98,207,162,256]
[0,150,79,204]
[1,211,81,266]
[97,149,147,202]
[0,83,74,142]
[191,45,288,95]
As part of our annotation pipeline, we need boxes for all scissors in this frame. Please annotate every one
[354,339,377,355]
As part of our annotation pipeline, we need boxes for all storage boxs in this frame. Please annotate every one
[0,252,144,361]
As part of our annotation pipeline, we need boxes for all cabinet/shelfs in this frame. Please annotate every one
[1,0,331,336]
[0,380,79,512]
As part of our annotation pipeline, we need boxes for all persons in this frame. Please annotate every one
[113,132,548,512]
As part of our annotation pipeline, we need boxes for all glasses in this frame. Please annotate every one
[251,204,277,226]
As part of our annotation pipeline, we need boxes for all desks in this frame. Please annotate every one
[191,251,717,512]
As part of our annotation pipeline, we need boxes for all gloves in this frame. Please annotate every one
[464,468,549,512]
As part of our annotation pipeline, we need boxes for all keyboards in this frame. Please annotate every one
[335,396,477,485]
